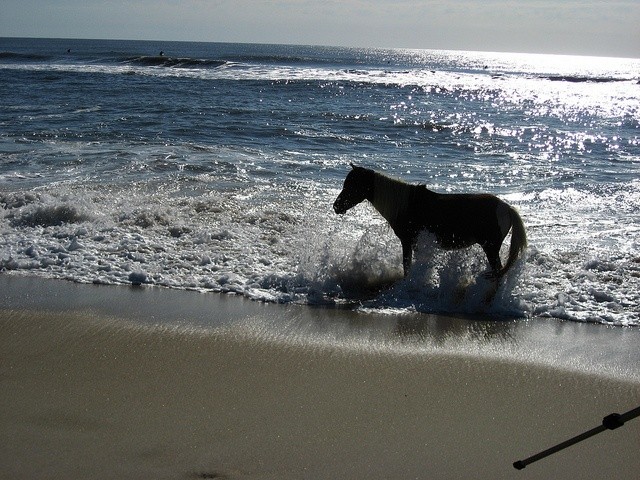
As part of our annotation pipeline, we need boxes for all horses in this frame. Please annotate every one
[332,162,528,283]
